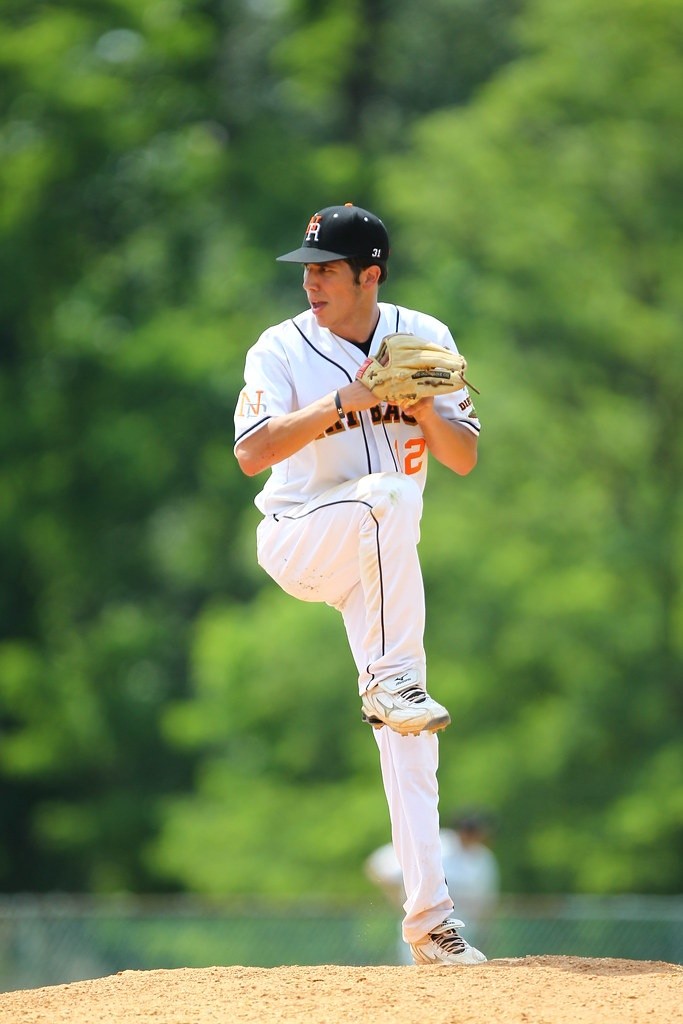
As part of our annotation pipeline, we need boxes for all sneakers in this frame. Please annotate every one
[410,918,488,965]
[362,672,451,736]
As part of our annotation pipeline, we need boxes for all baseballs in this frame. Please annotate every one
[396,395,422,411]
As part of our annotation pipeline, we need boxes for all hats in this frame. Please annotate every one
[276,203,388,262]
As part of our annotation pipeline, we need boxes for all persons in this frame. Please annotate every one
[233,202,488,966]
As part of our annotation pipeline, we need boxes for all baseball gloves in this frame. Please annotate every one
[355,331,469,404]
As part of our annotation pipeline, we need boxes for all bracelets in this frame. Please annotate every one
[332,389,345,419]
